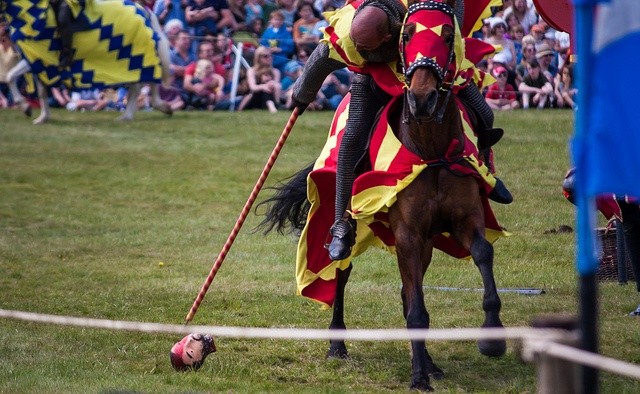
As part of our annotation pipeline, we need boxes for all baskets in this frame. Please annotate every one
[594,214,634,281]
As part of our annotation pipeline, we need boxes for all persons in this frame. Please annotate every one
[476,1,576,111]
[292,0,505,260]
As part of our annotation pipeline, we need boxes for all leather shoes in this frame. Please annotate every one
[488,177,513,203]
[328,229,355,259]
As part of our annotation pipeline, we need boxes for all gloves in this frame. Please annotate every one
[290,93,309,115]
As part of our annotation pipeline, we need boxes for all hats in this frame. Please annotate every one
[535,43,554,58]
[492,66,506,77]
[531,24,545,32]
[490,18,508,31]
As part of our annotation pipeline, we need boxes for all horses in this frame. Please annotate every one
[255,0,507,392]
[6,2,172,124]
